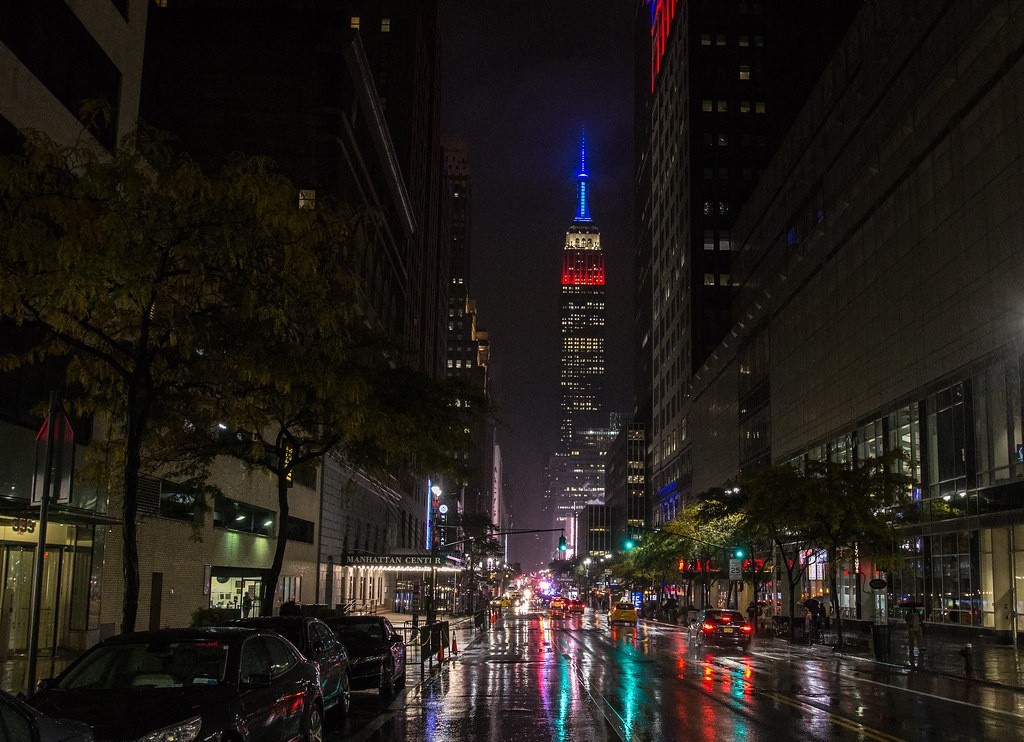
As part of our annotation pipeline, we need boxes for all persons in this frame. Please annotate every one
[809,603,827,624]
[593,595,607,612]
[704,603,714,609]
[728,602,734,610]
[745,601,766,627]
[639,597,696,619]
[243,592,252,619]
[277,596,296,616]
[905,608,927,652]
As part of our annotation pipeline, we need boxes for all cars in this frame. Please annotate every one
[531,578,585,614]
[688,608,754,652]
[323,615,406,701]
[0,628,324,742]
[606,602,638,628]
[225,618,351,718]
[490,576,529,606]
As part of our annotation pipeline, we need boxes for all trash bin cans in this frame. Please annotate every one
[669,609,677,623]
[868,625,892,662]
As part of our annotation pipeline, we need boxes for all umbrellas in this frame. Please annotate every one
[899,602,924,616]
[803,599,819,608]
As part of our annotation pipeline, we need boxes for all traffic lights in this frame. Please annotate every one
[559,536,566,550]
[622,536,634,551]
[733,548,746,561]
